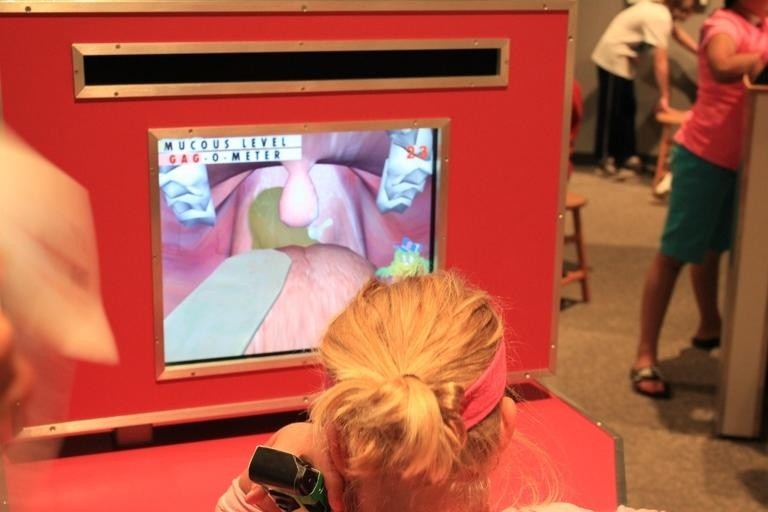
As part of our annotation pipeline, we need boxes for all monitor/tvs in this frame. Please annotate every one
[146,114,452,383]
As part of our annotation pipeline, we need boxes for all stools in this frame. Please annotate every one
[648,110,697,198]
[560,195,593,302]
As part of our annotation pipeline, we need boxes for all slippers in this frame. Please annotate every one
[629,367,671,398]
[694,333,719,351]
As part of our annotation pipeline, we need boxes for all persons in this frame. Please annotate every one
[630,0,767,402]
[567,80,585,179]
[213,266,665,511]
[1,314,33,443]
[590,0,698,181]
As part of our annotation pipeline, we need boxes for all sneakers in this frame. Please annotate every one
[625,158,646,174]
[595,161,633,180]
